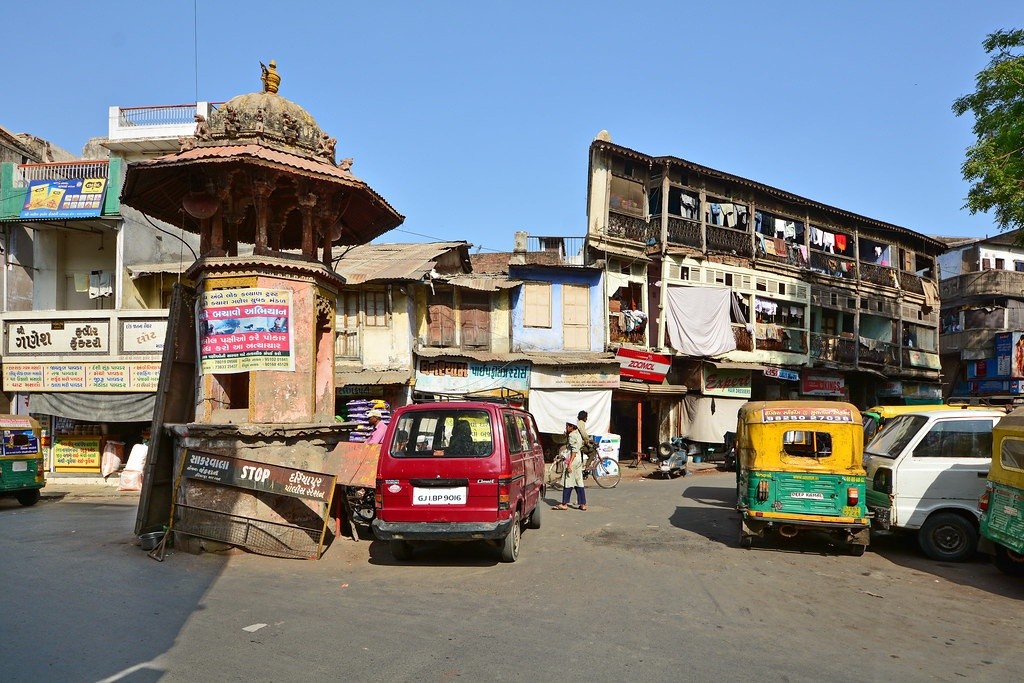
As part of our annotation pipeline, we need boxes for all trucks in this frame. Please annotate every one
[863,410,1007,562]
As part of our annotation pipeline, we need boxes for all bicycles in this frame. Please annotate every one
[346,487,376,521]
[549,444,621,491]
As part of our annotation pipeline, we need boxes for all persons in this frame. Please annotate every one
[552,419,587,510]
[577,410,596,470]
[395,430,408,451]
[357,410,388,495]
[416,436,431,450]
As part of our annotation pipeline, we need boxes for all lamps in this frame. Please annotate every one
[98,234,104,251]
[400,287,415,301]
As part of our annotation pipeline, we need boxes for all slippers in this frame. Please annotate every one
[579,506,586,510]
[552,505,566,510]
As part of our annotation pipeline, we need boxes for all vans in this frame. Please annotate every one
[371,387,545,562]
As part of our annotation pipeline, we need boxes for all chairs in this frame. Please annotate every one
[704,448,715,463]
[450,420,474,456]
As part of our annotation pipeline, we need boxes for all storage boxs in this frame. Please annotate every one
[590,434,621,476]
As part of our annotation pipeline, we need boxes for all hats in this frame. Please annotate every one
[566,418,578,425]
[366,410,381,418]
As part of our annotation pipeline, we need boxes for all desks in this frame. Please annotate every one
[629,452,646,470]
[52,434,121,473]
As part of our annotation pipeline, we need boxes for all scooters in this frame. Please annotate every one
[656,435,689,479]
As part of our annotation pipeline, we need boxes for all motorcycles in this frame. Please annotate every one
[0,414,47,507]
[976,406,1024,575]
[863,403,1010,448]
[735,401,876,556]
